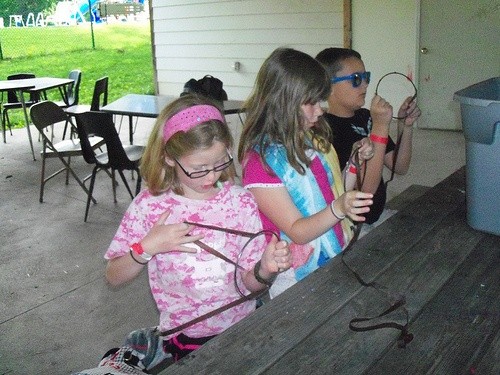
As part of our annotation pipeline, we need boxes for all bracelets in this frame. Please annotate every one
[369,133,389,146]
[329,199,345,220]
[131,243,153,260]
[349,166,357,174]
[129,251,147,266]
[253,260,276,285]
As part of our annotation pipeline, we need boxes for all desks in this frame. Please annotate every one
[102,92,249,145]
[9,15,22,27]
[0,81,36,94]
[159,162,500,375]
[20,76,74,102]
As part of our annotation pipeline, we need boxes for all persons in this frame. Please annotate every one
[237,47,375,301]
[104,94,291,362]
[315,47,422,239]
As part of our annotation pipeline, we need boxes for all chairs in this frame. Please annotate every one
[1,73,49,142]
[75,111,146,222]
[38,70,83,143]
[62,75,109,140]
[30,100,120,206]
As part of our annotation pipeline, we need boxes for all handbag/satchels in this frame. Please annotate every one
[197,75,223,100]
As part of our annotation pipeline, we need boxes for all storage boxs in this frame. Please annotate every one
[453,77,500,237]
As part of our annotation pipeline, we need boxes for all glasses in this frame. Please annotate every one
[330,71,370,87]
[169,148,233,179]
